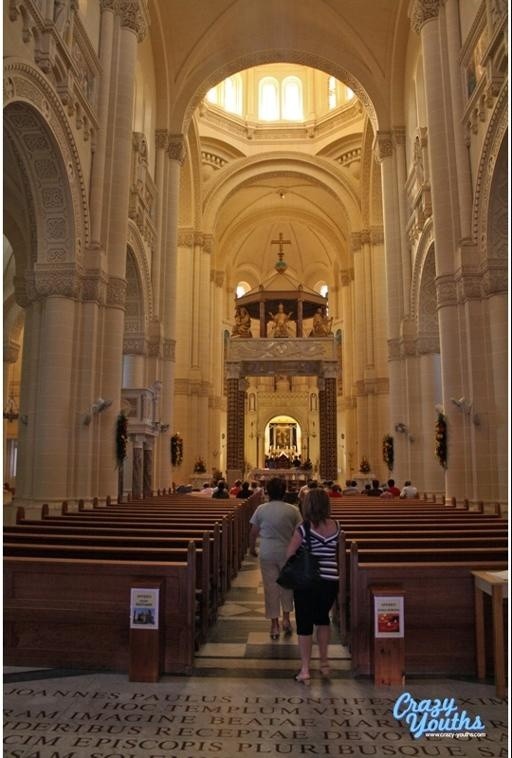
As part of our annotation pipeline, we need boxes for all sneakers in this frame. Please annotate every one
[283,619,291,635]
[270,632,280,639]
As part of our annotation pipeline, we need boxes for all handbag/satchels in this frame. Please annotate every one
[276,546,317,590]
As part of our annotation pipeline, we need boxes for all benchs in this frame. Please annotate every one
[319,492,508,680]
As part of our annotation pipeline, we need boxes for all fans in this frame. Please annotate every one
[394,422,415,441]
[448,395,480,426]
[81,398,114,425]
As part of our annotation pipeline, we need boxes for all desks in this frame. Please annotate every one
[470,571,508,700]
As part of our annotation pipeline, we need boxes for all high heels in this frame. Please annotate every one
[320,663,329,675]
[297,673,311,686]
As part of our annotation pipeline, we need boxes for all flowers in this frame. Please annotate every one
[433,413,448,469]
[382,434,394,470]
[172,431,184,465]
[117,412,128,462]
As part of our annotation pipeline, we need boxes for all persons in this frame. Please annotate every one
[309,306,334,337]
[269,303,294,337]
[230,307,252,338]
[249,476,304,639]
[287,489,342,686]
[177,456,420,500]
